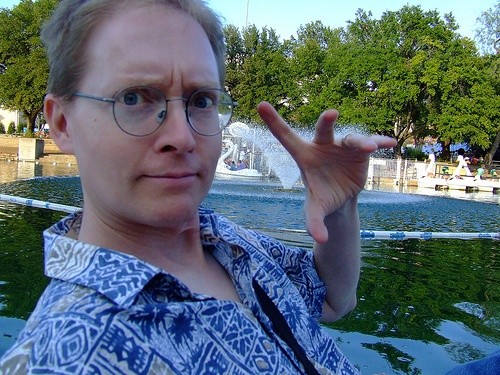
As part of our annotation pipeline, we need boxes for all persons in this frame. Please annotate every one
[0,1,401,374]
[472,157,477,171]
[229,159,245,171]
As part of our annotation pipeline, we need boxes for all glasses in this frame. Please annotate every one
[70,85,239,138]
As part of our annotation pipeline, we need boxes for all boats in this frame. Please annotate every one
[215,137,264,181]
[402,153,500,193]
